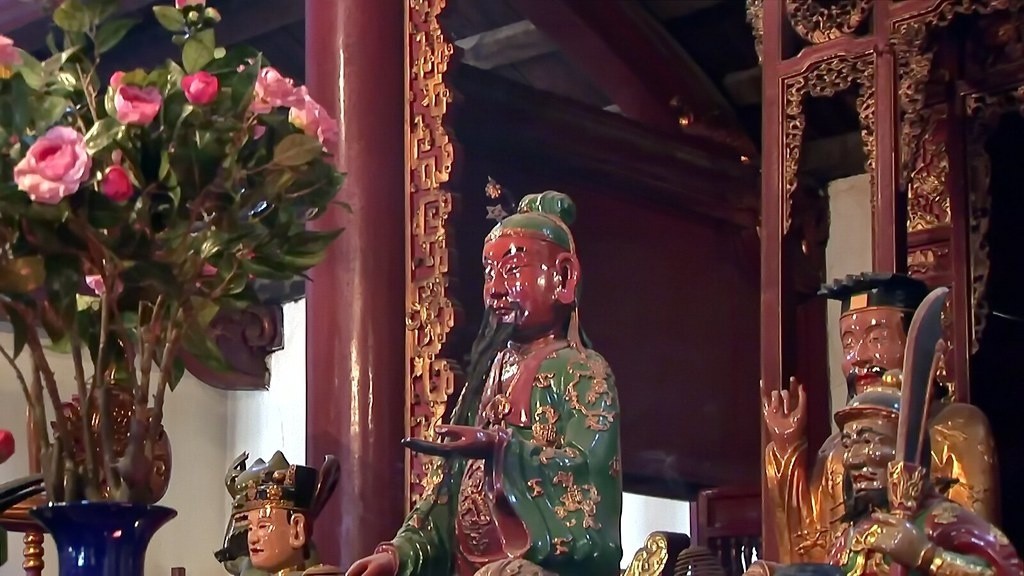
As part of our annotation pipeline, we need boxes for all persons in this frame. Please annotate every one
[244,464,318,576]
[757,272,1003,575]
[824,370,1021,576]
[345,190,626,576]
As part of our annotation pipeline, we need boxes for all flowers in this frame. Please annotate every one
[0,0,348,506]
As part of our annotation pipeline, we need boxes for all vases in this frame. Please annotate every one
[35,499,177,576]
